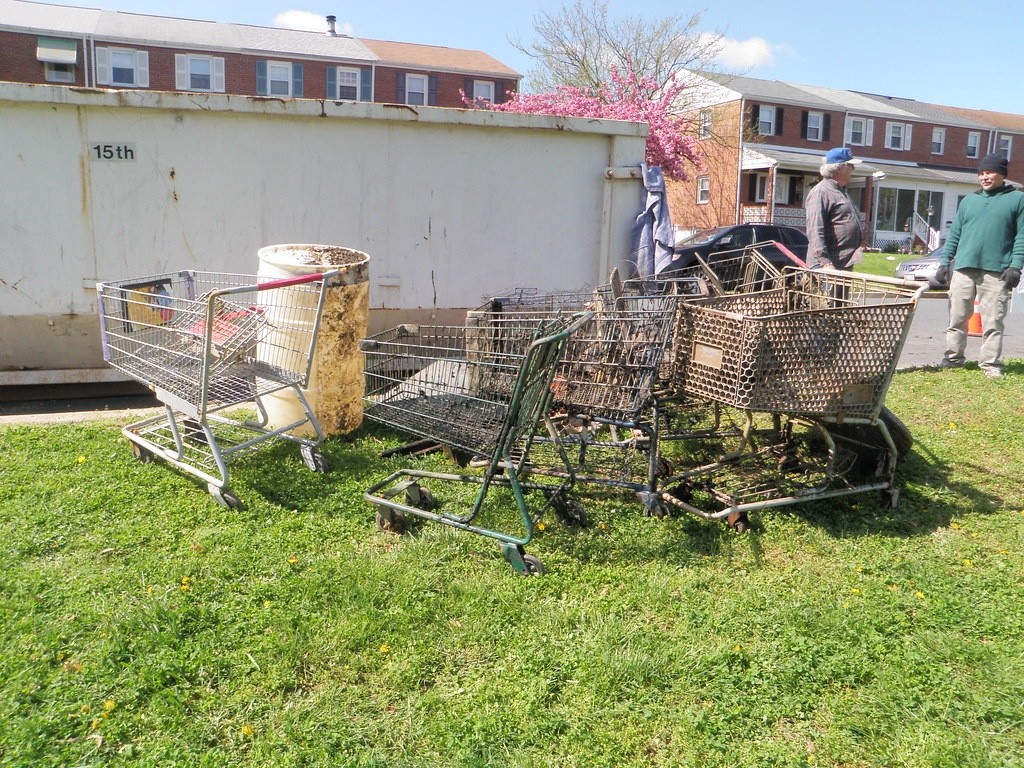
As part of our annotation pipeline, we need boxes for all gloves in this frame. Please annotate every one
[936,265,950,285]
[998,267,1022,288]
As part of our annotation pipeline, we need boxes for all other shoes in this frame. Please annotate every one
[981,368,1004,379]
[939,359,964,368]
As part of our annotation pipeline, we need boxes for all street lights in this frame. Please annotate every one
[924,205,937,253]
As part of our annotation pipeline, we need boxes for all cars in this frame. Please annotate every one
[649,221,813,295]
[894,244,962,288]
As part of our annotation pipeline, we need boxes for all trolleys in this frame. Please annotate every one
[480,240,931,539]
[348,307,604,574]
[96,269,349,507]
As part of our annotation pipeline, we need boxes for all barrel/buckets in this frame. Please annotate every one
[256,243,371,439]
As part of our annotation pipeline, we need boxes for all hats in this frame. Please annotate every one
[977,154,1008,179]
[826,148,863,164]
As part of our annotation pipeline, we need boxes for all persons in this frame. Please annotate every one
[934,155,1024,379]
[801,147,864,372]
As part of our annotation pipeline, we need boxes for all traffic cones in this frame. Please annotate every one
[968,292,983,337]
